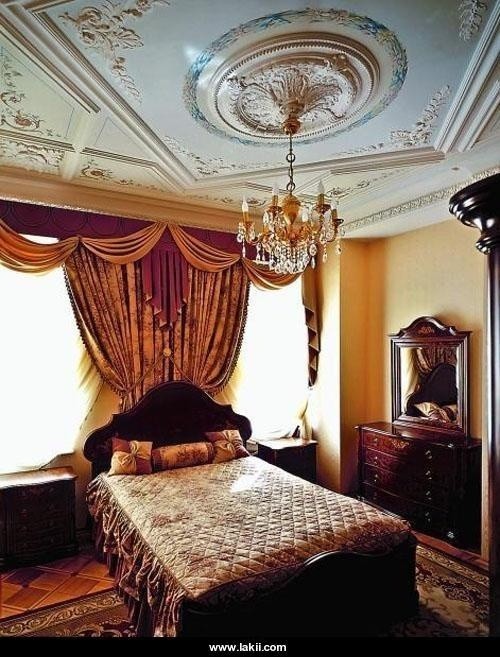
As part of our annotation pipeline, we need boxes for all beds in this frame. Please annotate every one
[82,379,419,637]
[404,363,457,425]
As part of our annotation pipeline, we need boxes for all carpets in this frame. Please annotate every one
[0,539,489,637]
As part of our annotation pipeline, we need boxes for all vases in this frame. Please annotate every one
[297,398,313,440]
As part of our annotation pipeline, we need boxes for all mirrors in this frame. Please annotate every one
[387,315,473,446]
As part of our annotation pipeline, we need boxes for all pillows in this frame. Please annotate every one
[413,401,458,423]
[106,429,251,478]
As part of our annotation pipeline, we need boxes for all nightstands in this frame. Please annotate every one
[256,436,319,485]
[0,466,80,570]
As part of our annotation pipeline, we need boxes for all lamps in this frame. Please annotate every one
[235,115,346,275]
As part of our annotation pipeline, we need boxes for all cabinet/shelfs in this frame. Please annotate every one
[351,420,482,555]
